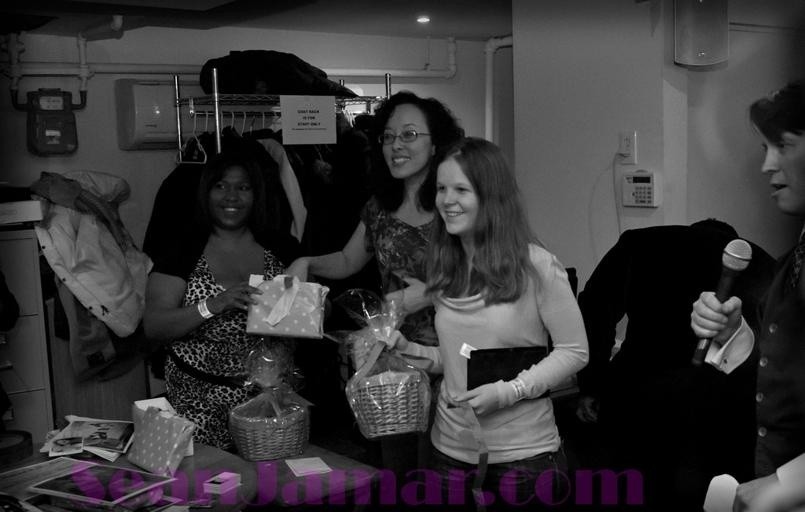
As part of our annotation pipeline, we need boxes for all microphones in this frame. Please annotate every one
[691,239,753,367]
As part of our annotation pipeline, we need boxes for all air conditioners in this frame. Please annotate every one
[116,78,277,149]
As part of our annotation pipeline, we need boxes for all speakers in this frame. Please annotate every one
[674,0,730,66]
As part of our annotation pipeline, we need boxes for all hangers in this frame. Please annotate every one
[176,113,277,165]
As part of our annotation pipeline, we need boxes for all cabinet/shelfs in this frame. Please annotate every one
[0,232,60,470]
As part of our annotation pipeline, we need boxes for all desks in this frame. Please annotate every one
[1,446,387,512]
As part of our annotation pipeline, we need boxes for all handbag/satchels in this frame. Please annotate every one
[127,405,196,478]
[246,273,331,340]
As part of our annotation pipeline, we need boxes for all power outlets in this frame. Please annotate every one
[617,129,638,164]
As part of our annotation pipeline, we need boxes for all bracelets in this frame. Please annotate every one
[197,299,216,320]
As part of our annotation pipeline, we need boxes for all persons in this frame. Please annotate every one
[378,130,594,474]
[568,217,780,511]
[281,87,469,478]
[139,143,324,464]
[53,435,83,447]
[687,70,805,511]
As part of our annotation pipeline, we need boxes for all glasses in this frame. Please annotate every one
[378,129,432,145]
